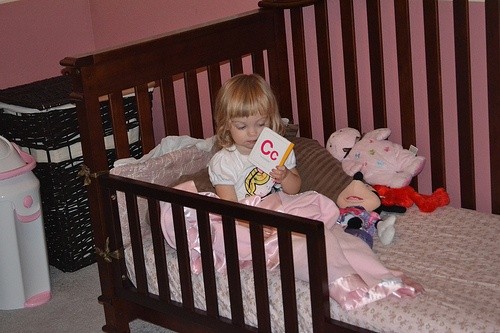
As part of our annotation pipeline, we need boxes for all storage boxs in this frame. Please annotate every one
[1,74,154,272]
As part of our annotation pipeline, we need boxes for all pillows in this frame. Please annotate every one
[144,124,353,227]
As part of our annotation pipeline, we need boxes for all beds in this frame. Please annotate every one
[59,1,500,333]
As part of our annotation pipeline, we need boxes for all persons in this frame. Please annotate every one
[209,74,302,203]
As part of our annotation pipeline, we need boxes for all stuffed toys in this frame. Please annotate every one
[337,171,395,250]
